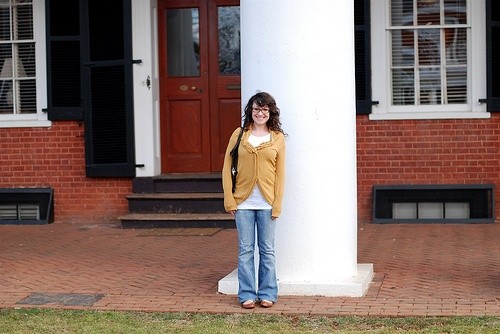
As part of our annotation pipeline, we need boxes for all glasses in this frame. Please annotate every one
[252,108,270,114]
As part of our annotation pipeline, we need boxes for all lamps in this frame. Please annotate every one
[0,57,27,108]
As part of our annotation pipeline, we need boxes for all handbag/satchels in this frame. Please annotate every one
[230,127,244,194]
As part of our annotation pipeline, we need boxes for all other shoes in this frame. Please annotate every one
[242,300,255,309]
[261,300,274,307]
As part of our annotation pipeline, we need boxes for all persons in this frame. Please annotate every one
[221,92,286,309]
